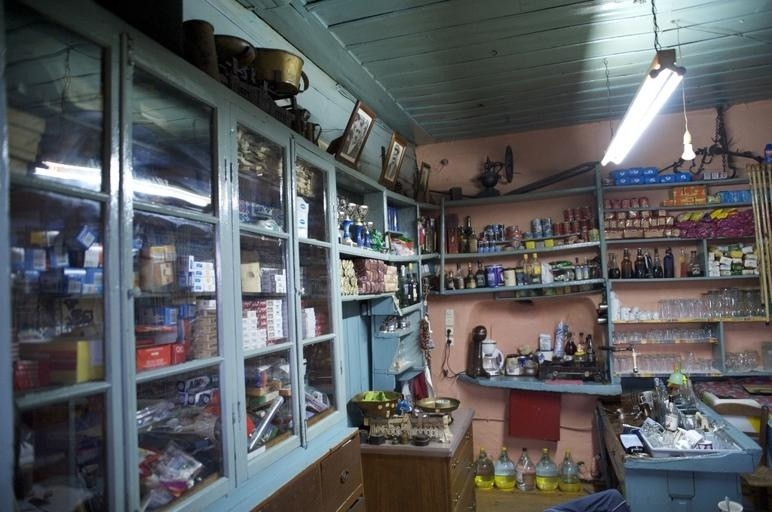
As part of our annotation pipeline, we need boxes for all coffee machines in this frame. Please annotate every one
[481,340,504,376]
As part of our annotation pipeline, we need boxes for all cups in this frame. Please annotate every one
[609,285,766,321]
[611,327,713,374]
[504,353,538,376]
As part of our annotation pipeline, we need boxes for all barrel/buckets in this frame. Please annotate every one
[473,447,495,491]
[493,448,517,491]
[512,446,535,493]
[560,448,581,493]
[534,447,559,491]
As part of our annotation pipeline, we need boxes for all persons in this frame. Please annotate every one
[386,144,401,179]
[343,119,366,159]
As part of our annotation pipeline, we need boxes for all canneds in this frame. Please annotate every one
[522,206,600,249]
[484,264,524,287]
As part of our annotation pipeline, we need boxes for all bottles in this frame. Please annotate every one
[447,259,487,289]
[417,215,507,253]
[383,316,412,333]
[502,267,523,287]
[556,320,595,363]
[605,245,703,279]
[398,264,421,308]
[652,376,733,449]
[473,446,581,491]
[391,430,410,446]
[521,252,542,284]
[575,256,597,281]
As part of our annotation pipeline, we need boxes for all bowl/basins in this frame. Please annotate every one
[214,34,254,66]
[254,46,309,94]
